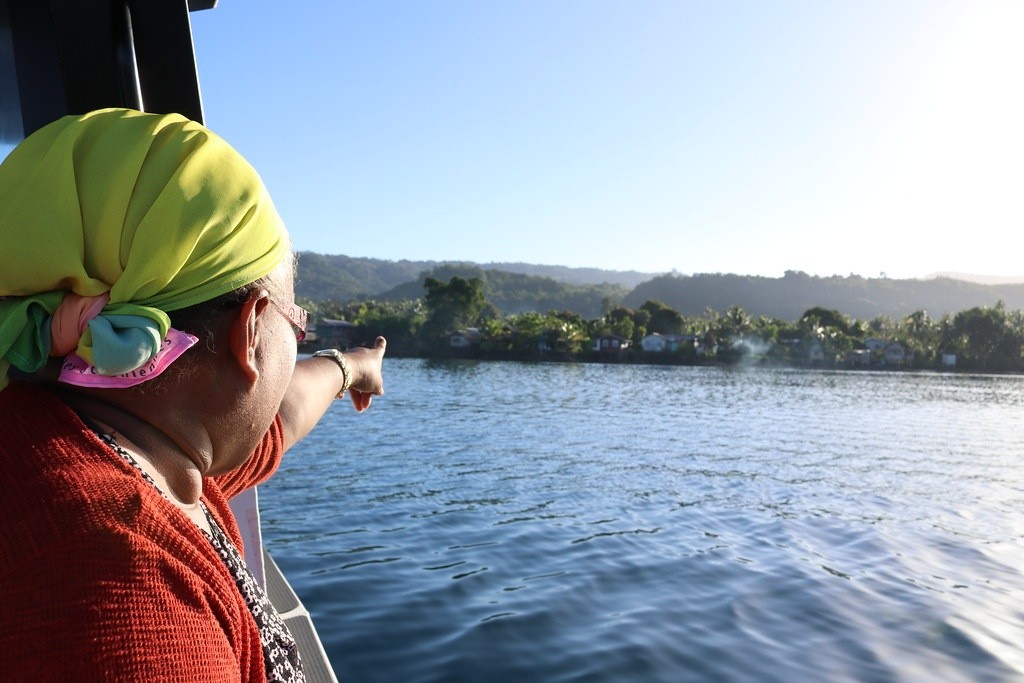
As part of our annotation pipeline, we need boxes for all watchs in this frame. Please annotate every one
[313,348,352,399]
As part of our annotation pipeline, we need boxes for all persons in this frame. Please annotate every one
[1,107,386,683]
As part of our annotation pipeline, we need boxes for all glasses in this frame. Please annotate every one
[223,285,312,342]
